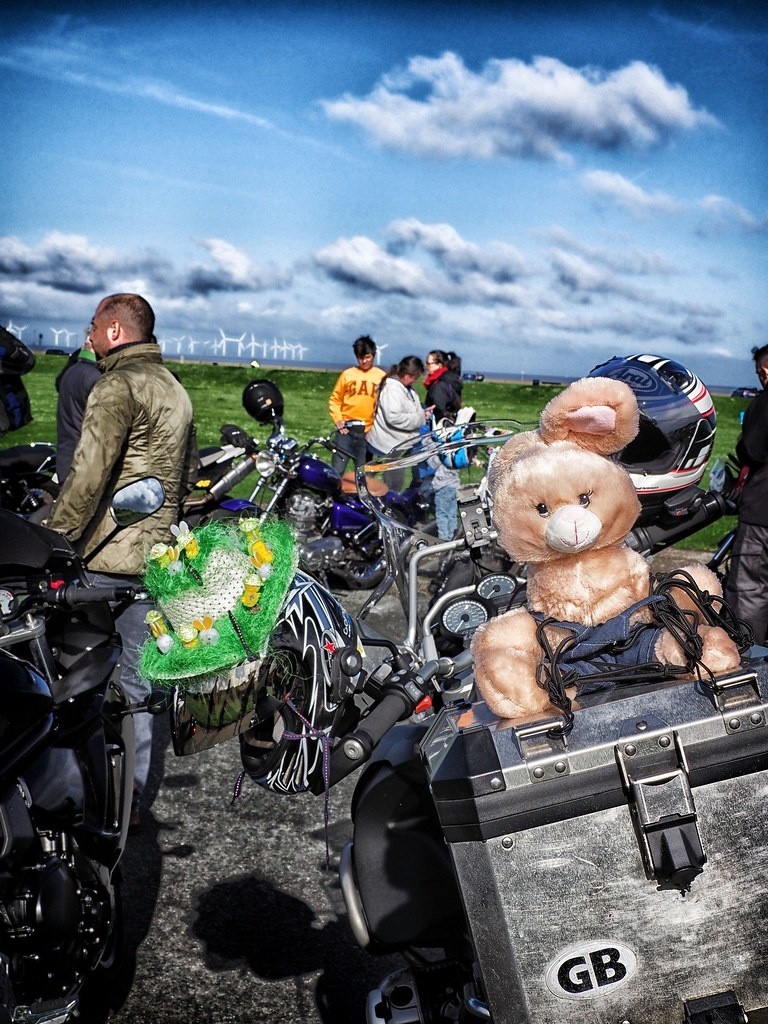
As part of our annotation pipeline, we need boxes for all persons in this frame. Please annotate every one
[726,344,768,648]
[42,293,200,836]
[327,333,463,541]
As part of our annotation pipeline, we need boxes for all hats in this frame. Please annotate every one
[137,520,299,677]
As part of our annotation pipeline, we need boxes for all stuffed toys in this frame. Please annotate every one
[469,376,742,719]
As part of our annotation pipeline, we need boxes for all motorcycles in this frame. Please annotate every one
[0,447,768,1024]
[181,379,430,592]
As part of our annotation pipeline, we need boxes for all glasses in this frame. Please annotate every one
[426,361,439,366]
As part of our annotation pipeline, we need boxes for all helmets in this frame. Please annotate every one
[242,380,284,422]
[588,353,716,495]
[239,567,356,794]
[432,406,476,469]
